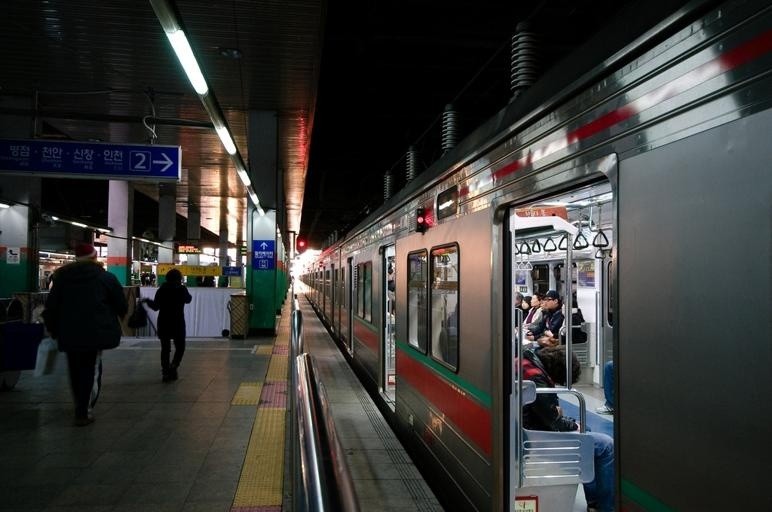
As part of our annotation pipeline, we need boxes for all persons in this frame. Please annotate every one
[44,239,128,425]
[141,269,192,383]
[515,291,614,512]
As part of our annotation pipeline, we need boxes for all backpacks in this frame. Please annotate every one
[534,346,581,386]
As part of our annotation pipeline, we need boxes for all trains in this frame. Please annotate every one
[298,1,772,511]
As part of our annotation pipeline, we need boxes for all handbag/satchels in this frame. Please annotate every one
[127,301,147,328]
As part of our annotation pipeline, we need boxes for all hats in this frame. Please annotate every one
[544,290,559,297]
[76,244,96,261]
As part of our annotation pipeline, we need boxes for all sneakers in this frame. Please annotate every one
[587,504,598,512]
[76,417,94,425]
[596,405,615,415]
[162,367,177,382]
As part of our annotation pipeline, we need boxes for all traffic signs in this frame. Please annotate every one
[0,138,183,183]
[252,240,275,272]
